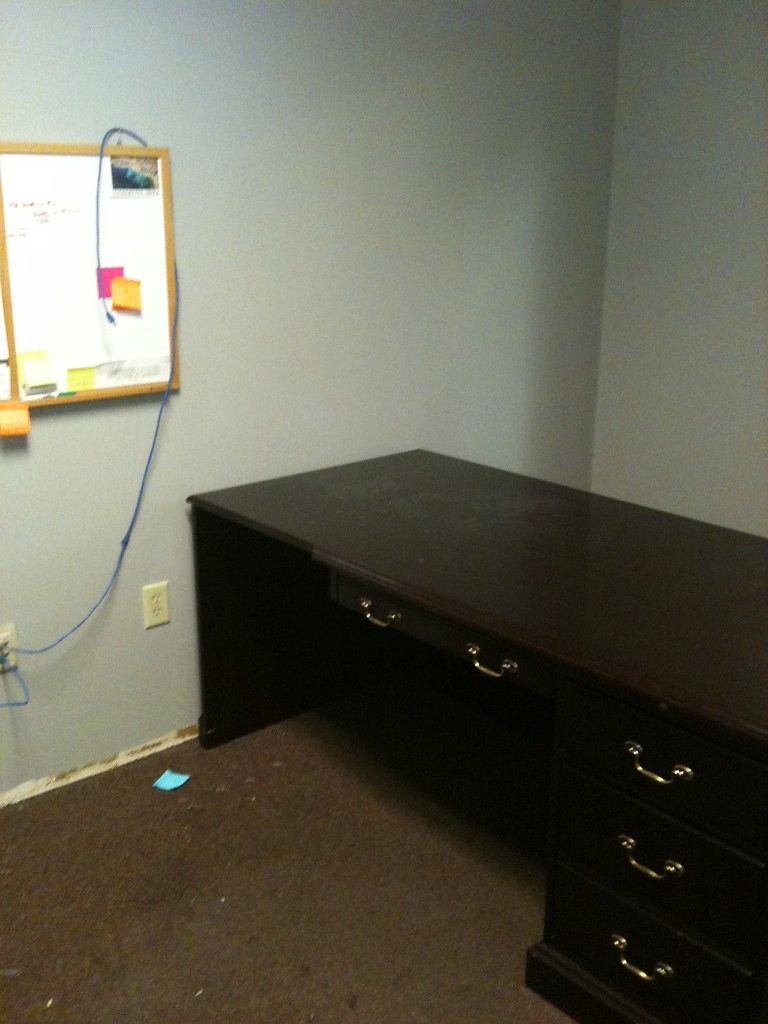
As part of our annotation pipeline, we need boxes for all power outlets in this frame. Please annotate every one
[142,580,171,630]
[0,624,18,672]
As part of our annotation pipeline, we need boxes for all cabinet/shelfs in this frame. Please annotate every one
[186,448,767,1023]
